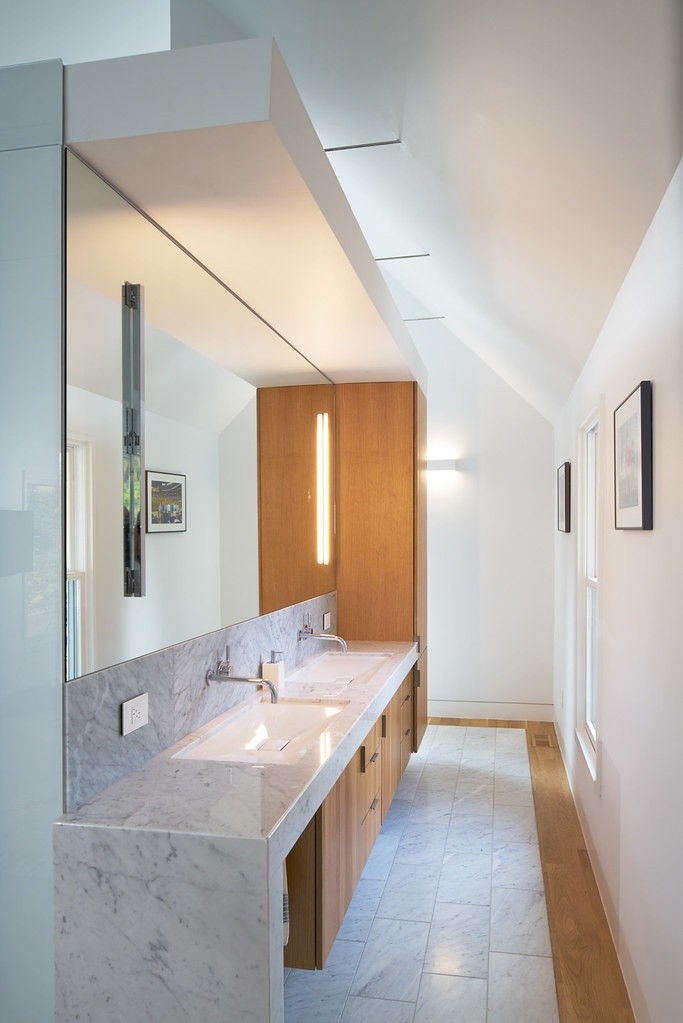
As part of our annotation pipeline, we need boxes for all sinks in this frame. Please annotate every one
[168,698,353,766]
[285,651,393,687]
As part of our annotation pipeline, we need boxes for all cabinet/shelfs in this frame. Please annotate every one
[255,378,339,611]
[340,379,431,754]
[283,719,383,973]
[390,669,414,779]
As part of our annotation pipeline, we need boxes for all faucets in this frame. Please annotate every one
[298,627,348,653]
[206,669,279,704]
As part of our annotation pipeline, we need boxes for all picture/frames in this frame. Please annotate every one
[611,379,654,533]
[555,460,573,535]
[144,469,187,535]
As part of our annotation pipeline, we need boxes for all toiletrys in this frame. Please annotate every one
[262,650,285,691]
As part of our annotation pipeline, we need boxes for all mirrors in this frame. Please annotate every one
[59,138,340,687]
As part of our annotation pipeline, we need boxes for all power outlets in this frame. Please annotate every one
[119,691,150,738]
[322,611,331,630]
